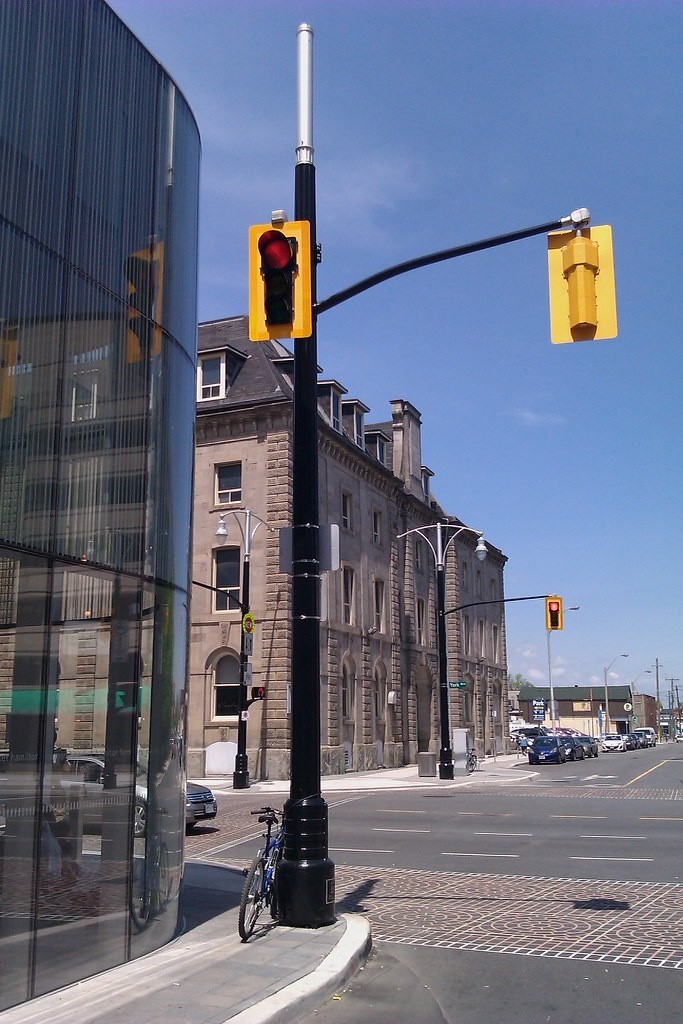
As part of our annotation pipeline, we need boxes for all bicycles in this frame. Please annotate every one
[238,806,287,939]
[465,748,478,773]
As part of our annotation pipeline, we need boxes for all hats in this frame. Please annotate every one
[520,734,525,737]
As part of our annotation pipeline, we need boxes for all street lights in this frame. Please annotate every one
[667,690,675,739]
[215,508,276,789]
[396,522,489,779]
[546,605,582,731]
[604,653,630,732]
[630,670,652,729]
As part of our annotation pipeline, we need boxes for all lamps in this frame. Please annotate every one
[477,656,485,664]
[367,627,378,634]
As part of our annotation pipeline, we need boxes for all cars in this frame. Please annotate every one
[509,725,657,760]
[527,736,567,766]
[1,745,220,839]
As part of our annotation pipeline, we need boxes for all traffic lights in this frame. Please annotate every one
[123,241,166,364]
[545,597,563,630]
[247,218,314,343]
[251,687,266,698]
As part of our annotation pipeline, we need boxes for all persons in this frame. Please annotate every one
[519,734,528,756]
[51,731,72,788]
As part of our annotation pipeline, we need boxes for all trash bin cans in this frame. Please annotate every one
[418,752,437,777]
